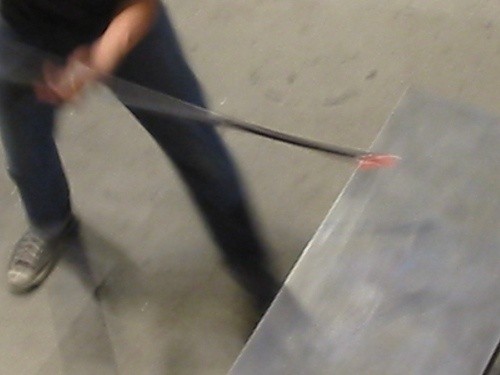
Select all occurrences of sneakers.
[7,214,83,293]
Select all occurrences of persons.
[0,0,281,322]
